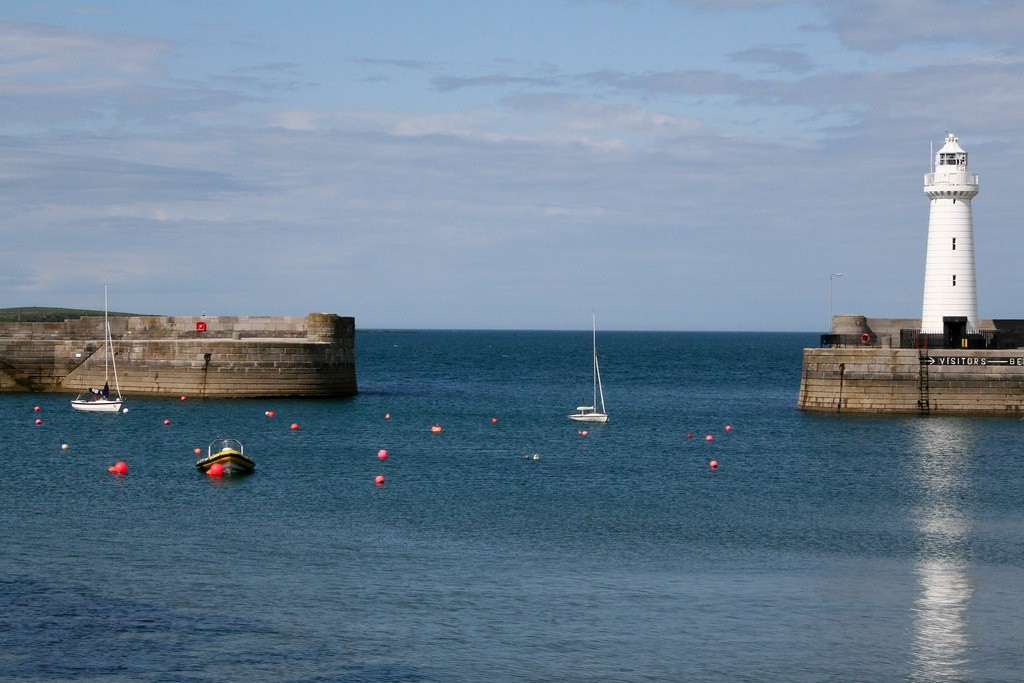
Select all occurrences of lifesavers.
[861,334,870,345]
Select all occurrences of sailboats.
[568,313,609,423]
[69,284,125,414]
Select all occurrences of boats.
[195,437,257,480]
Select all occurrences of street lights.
[829,272,845,333]
